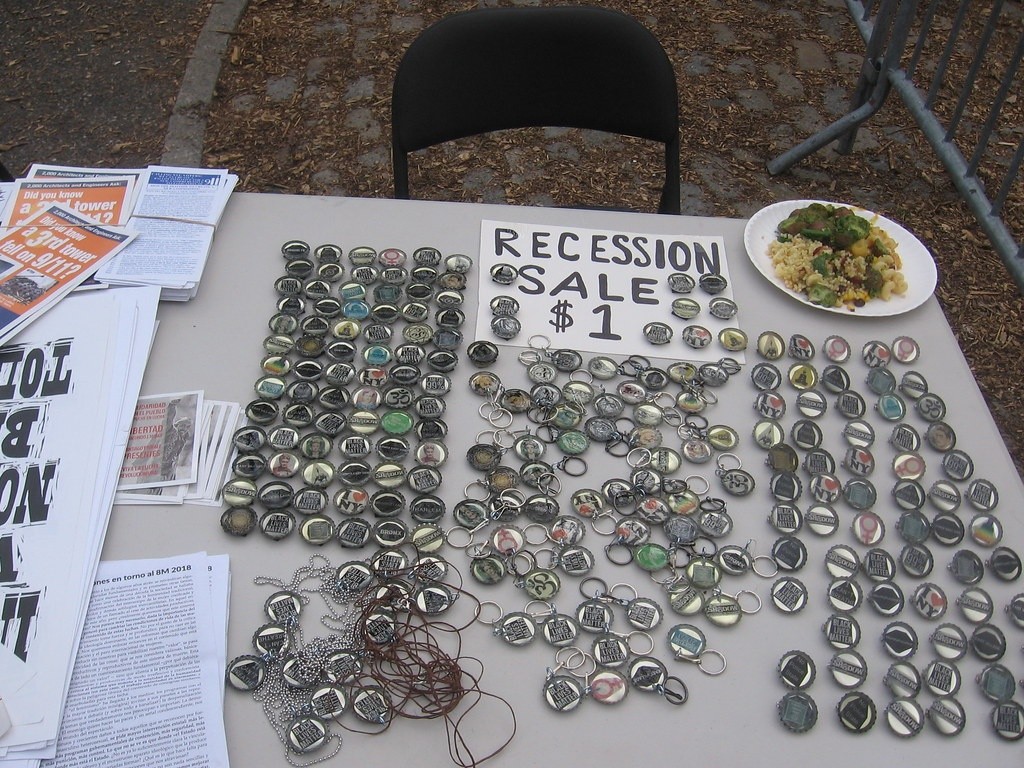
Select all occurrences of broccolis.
[778,203,907,307]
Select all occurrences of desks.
[74,191,1024,767]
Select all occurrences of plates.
[744,199,938,317]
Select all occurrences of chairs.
[392,5,682,217]
